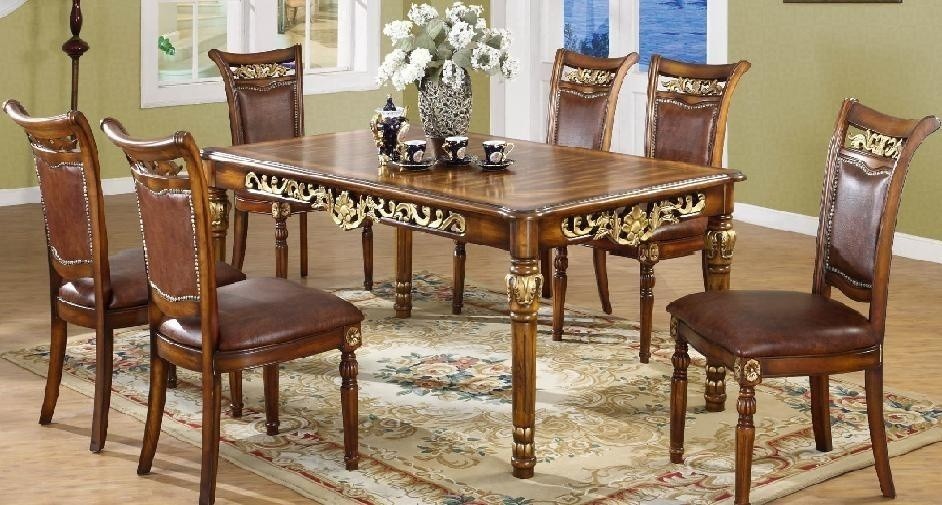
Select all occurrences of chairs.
[208,44,376,291]
[549,54,753,365]
[451,48,640,314]
[665,95,942,505]
[98,115,365,505]
[1,99,248,453]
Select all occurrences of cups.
[396,139,426,165]
[482,140,514,163]
[442,137,469,162]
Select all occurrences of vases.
[416,66,473,156]
[375,1,519,89]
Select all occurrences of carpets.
[0,269,942,505]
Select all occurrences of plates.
[392,159,436,171]
[434,154,478,167]
[476,159,515,171]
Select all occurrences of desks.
[199,120,748,479]
